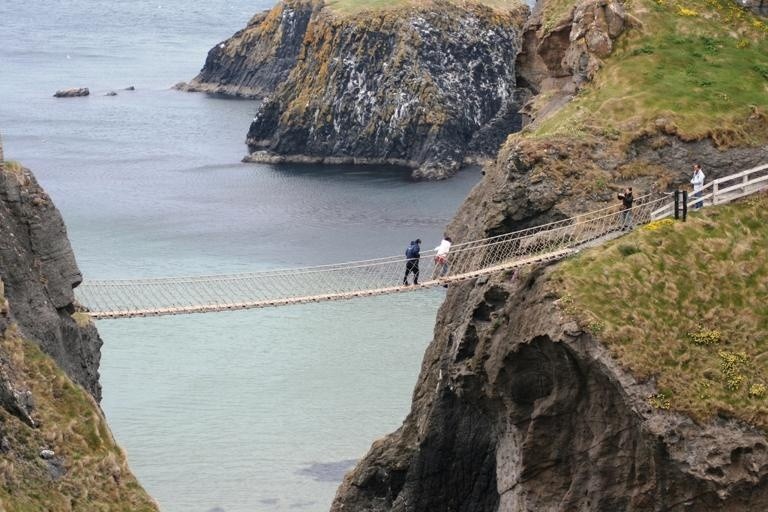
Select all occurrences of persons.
[690,164,706,208]
[618,187,633,231]
[432,237,452,280]
[403,239,422,286]
